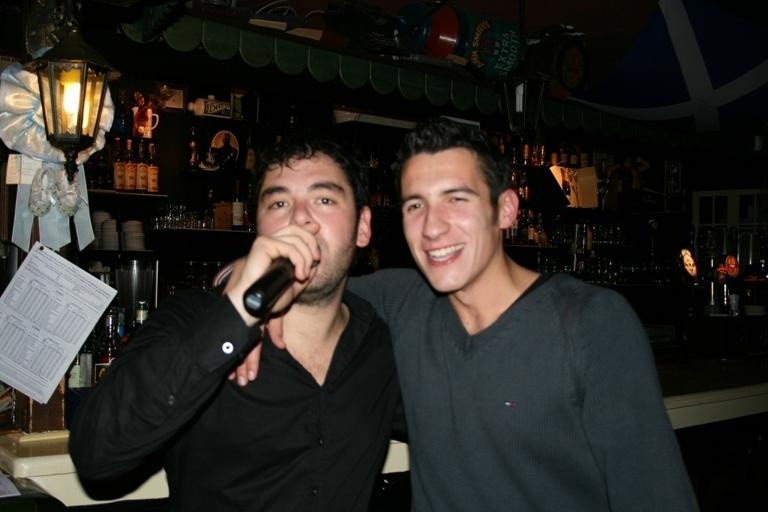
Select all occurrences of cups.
[744,305,765,316]
[115,260,230,328]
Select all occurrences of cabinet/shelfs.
[2,77,332,424]
[334,112,691,293]
[692,188,768,262]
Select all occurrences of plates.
[91,211,145,253]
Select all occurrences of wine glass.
[159,84,169,110]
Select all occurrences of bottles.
[66,301,149,389]
[85,94,633,279]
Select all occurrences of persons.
[219,116,699,511]
[67,136,409,511]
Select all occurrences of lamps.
[24,0,115,182]
[496,1,551,146]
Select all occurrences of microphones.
[243,257,321,318]
